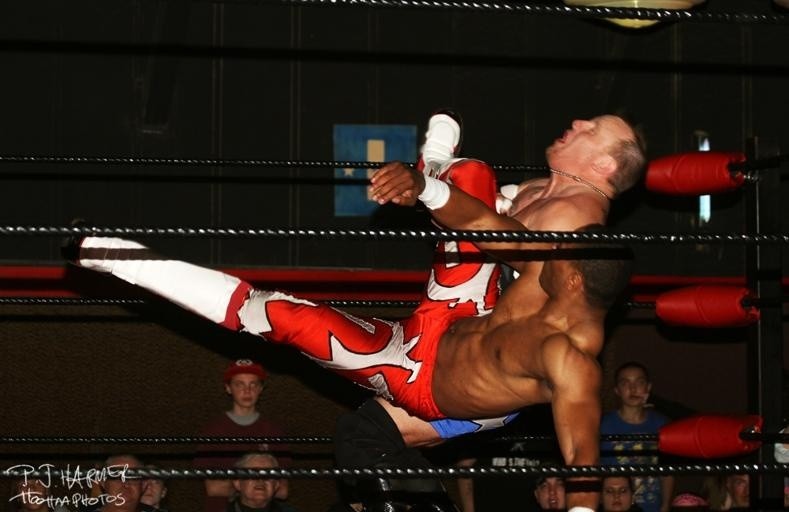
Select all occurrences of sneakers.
[415,106,465,192]
[59,211,111,278]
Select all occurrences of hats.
[224,358,267,384]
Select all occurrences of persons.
[50,106,647,512]
[5,477,50,511]
[673,466,753,510]
[597,360,677,469]
